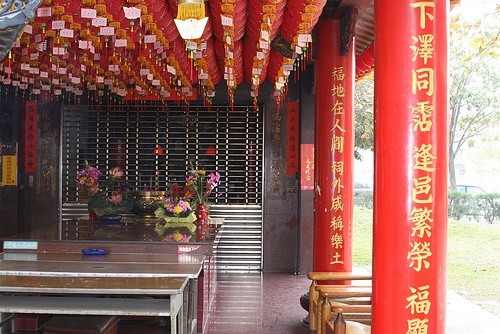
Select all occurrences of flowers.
[75,163,122,213]
[185,163,218,204]
[163,231,191,242]
[159,183,192,213]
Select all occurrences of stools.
[43,315,118,334]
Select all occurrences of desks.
[0,218,226,334]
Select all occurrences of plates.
[81,248,111,255]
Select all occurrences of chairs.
[306,270,371,334]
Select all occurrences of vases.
[154,208,197,223]
[99,213,121,222]
[195,204,208,219]
